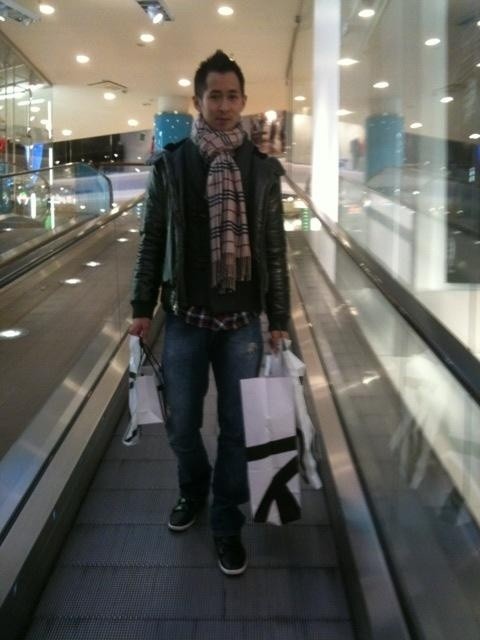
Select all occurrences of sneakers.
[213,532,247,576]
[168,499,205,530]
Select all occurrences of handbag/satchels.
[238,348,300,528]
[135,364,166,425]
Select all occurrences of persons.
[128,48,291,577]
